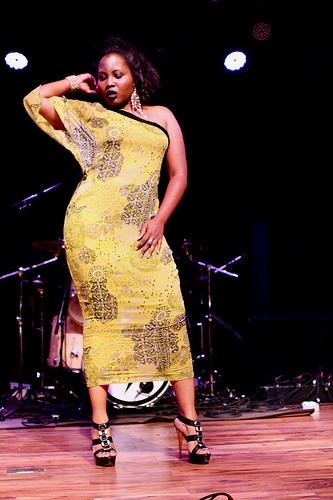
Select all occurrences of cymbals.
[33,241,65,253]
[22,279,62,288]
[169,242,221,256]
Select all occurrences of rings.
[147,239,153,244]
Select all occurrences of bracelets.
[65,74,80,91]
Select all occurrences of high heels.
[90,419,116,466]
[173,415,211,464]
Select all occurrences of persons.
[22,38,212,467]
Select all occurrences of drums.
[105,378,170,410]
[48,311,84,370]
[68,280,84,326]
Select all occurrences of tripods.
[184,255,243,406]
[1,253,82,420]
